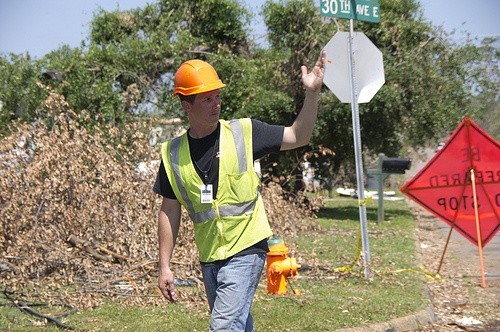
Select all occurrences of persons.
[152,48,328,332]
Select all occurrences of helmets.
[173,59,227,97]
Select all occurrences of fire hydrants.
[266,232,302,297]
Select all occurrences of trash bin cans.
[366,168,380,191]
